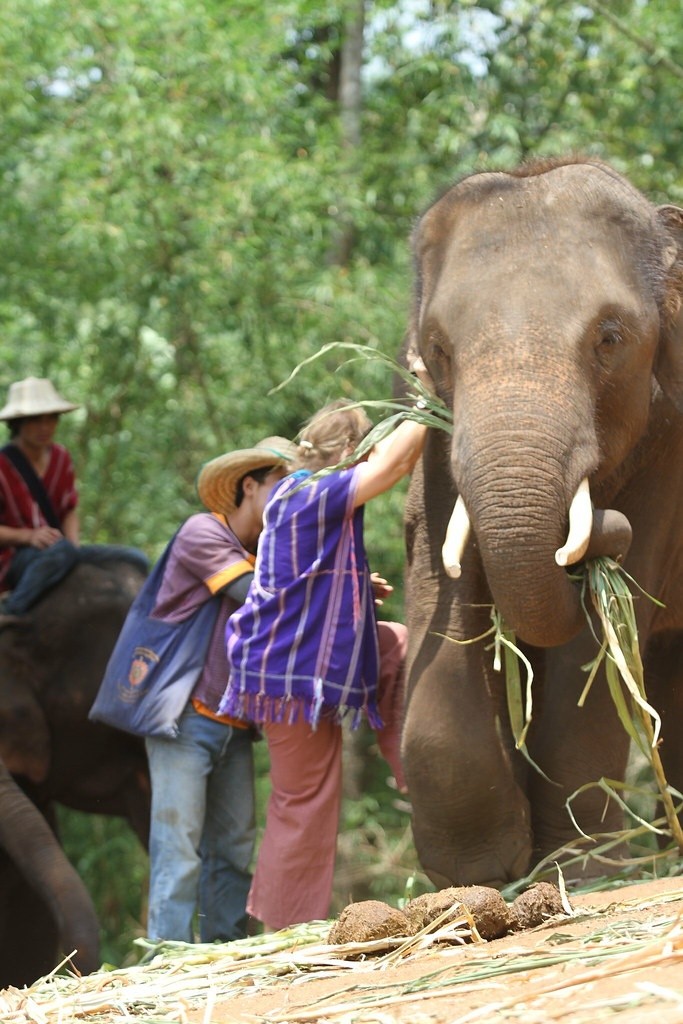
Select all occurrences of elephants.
[400,164,683,899]
[1,540,151,986]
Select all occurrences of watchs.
[415,397,432,410]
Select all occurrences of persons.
[145,435,394,966]
[219,358,437,937]
[0,377,149,626]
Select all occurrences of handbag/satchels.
[87,529,224,738]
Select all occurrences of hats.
[0,377,77,421]
[196,436,300,519]
